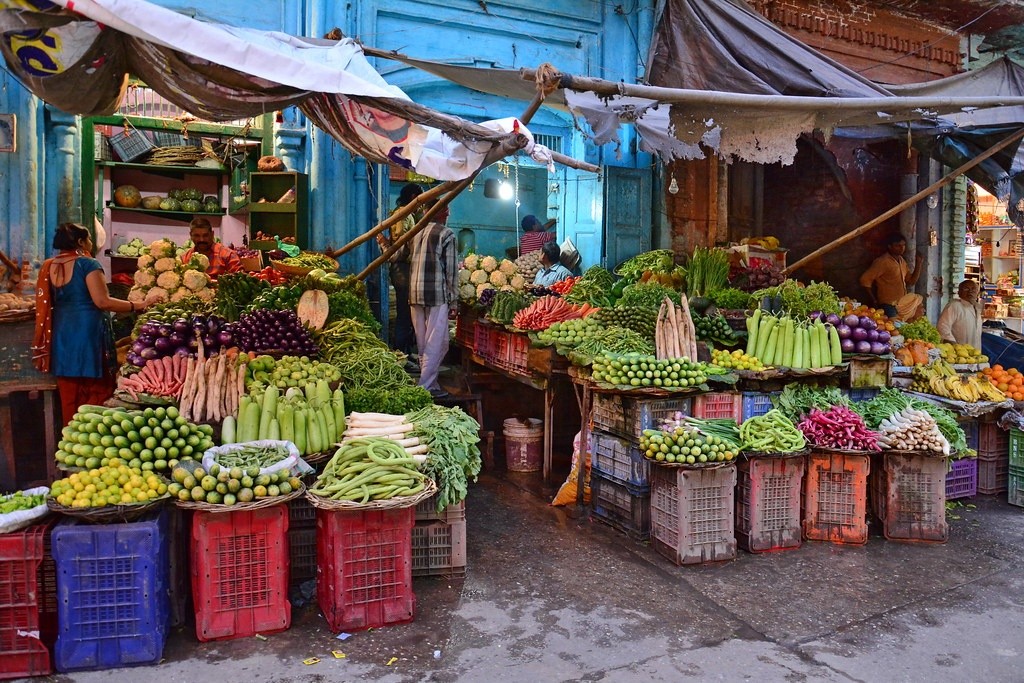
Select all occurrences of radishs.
[769,382,977,474]
[330,404,483,514]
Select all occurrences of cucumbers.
[54,403,216,469]
[639,428,739,464]
[595,303,747,345]
[590,350,707,387]
[168,465,302,505]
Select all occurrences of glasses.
[959,287,979,292]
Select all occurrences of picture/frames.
[0,113,17,153]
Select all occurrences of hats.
[892,292,923,323]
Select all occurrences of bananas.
[910,359,1007,405]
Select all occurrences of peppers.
[240,285,302,313]
[561,264,635,307]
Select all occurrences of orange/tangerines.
[976,364,1024,403]
[51,466,168,508]
[711,349,766,375]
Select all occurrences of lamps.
[668,171,679,195]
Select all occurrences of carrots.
[116,354,187,402]
[512,295,602,331]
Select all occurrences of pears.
[935,343,988,363]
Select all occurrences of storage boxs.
[238,249,264,272]
[0,295,1023,679]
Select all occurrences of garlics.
[656,409,749,451]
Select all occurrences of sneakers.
[430,389,448,399]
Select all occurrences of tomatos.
[247,265,289,285]
[547,275,583,295]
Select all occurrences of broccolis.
[454,253,525,300]
[118,237,219,305]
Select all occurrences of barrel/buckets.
[503,418,542,472]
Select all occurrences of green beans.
[320,315,412,390]
[216,445,290,468]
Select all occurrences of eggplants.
[807,311,892,356]
[126,307,319,366]
[527,284,560,298]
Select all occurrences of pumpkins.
[113,183,221,213]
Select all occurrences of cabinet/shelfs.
[248,170,310,251]
[96,160,228,217]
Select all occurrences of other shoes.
[407,352,417,363]
[403,361,421,373]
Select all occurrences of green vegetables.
[617,244,751,309]
[344,386,435,415]
[327,290,382,332]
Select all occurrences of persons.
[179,218,244,291]
[937,280,984,356]
[33,222,162,433]
[387,183,426,374]
[858,232,925,317]
[375,197,460,402]
[519,214,558,259]
[533,242,575,290]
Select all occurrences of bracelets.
[127,301,135,313]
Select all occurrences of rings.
[376,236,378,239]
